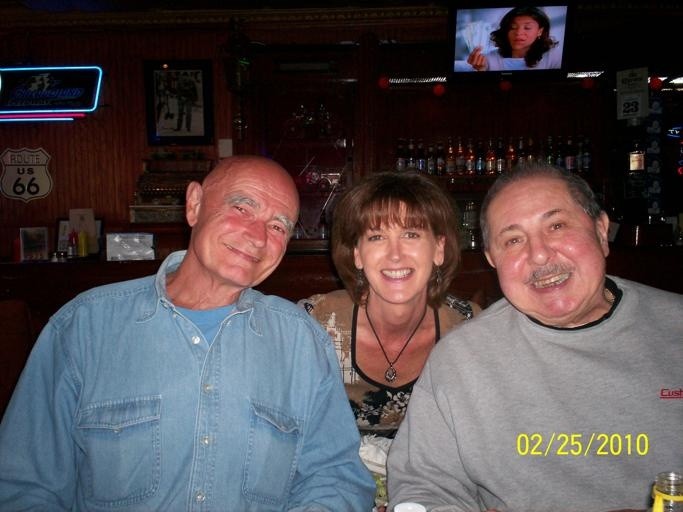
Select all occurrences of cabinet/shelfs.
[362,54,683,271]
[226,36,362,273]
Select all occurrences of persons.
[299,168,483,447]
[466,7,562,70]
[385,161,683,512]
[0,152,378,512]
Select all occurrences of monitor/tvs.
[448,0,571,73]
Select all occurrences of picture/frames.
[19,226,48,262]
[54,218,104,257]
[143,56,216,145]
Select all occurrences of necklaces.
[365,292,427,382]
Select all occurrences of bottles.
[628,118,645,175]
[50,228,87,263]
[461,200,480,249]
[394,133,591,178]
